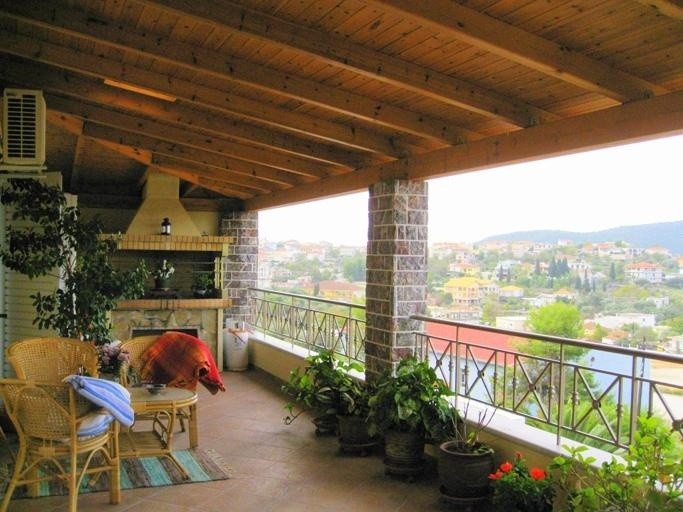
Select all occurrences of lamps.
[158,216,172,237]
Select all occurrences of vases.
[154,280,172,289]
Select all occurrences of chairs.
[0,332,200,511]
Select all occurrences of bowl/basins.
[145,383,166,395]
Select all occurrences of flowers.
[151,259,178,279]
[485,448,559,512]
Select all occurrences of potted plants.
[190,272,213,298]
[276,348,500,511]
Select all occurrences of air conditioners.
[0,87,47,172]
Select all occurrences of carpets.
[0,447,232,502]
